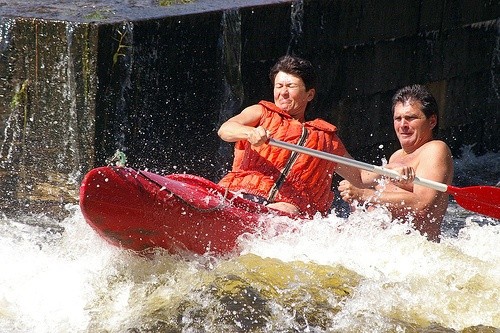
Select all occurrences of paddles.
[266,136,496,218]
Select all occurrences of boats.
[80,166,313,255]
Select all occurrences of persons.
[216,52,415,236]
[332,82,455,243]
